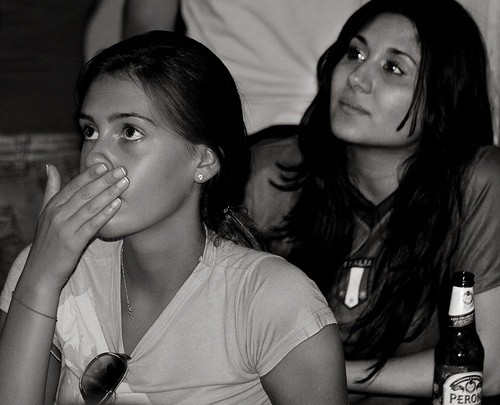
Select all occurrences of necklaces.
[120,250,142,340]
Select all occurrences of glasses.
[79,352,131,405]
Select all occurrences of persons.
[0,29,352,405]
[122,0,500,405]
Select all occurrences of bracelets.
[12,291,57,322]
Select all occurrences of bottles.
[433,271,485,405]
